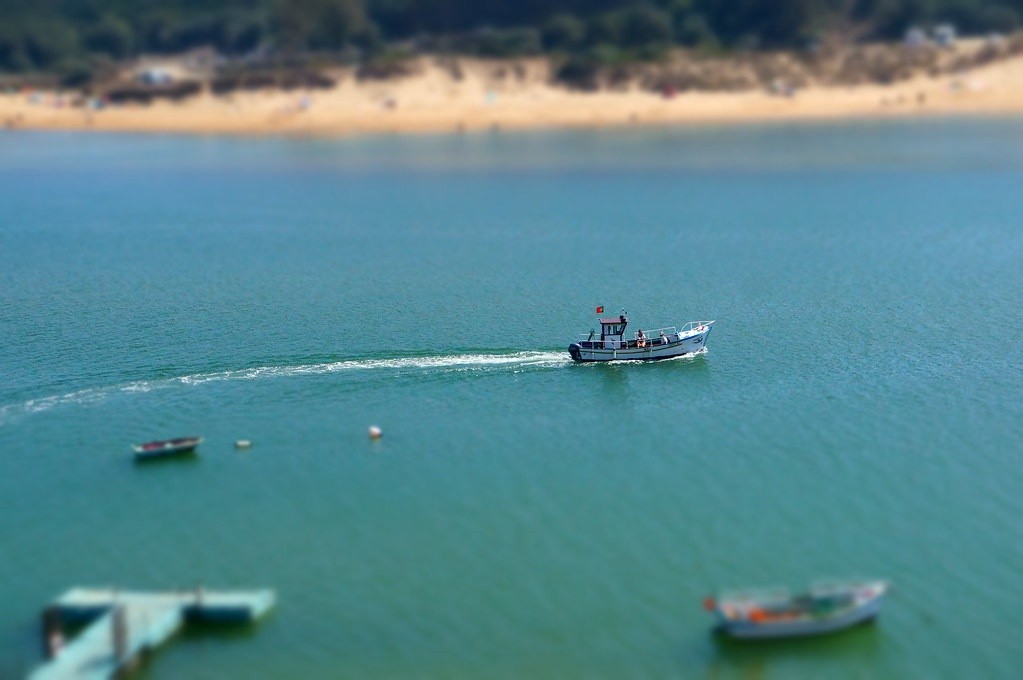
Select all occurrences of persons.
[660,331,668,344]
[636,329,646,348]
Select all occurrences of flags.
[596,305,604,313]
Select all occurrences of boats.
[708,576,891,637]
[567,311,715,365]
[132,435,205,458]
